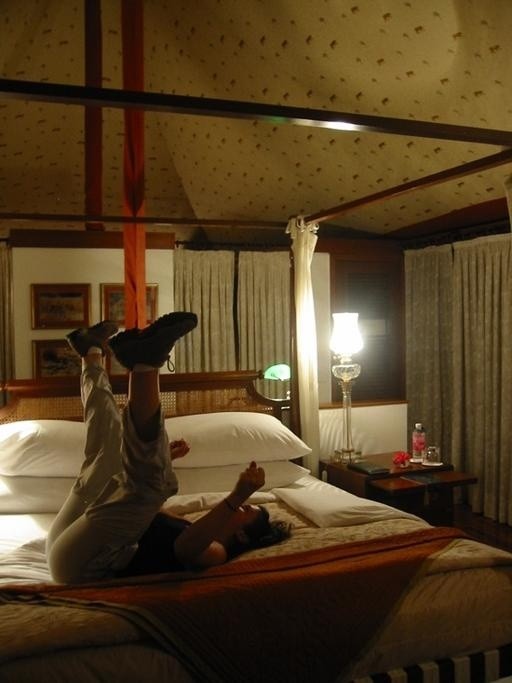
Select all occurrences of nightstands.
[318,451,478,527]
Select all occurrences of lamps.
[329,312,365,465]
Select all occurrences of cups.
[422,447,441,464]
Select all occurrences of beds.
[0,370,512,682]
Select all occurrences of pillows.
[0,420,89,477]
[0,476,79,515]
[172,460,311,495]
[165,411,313,468]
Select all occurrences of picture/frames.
[30,283,92,330]
[100,283,159,328]
[32,338,82,379]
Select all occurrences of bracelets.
[223,497,237,513]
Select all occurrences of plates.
[408,458,427,463]
[422,462,444,467]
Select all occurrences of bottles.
[412,423,425,459]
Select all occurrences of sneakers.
[64,317,117,356]
[110,312,201,371]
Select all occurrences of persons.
[44,311,293,583]
